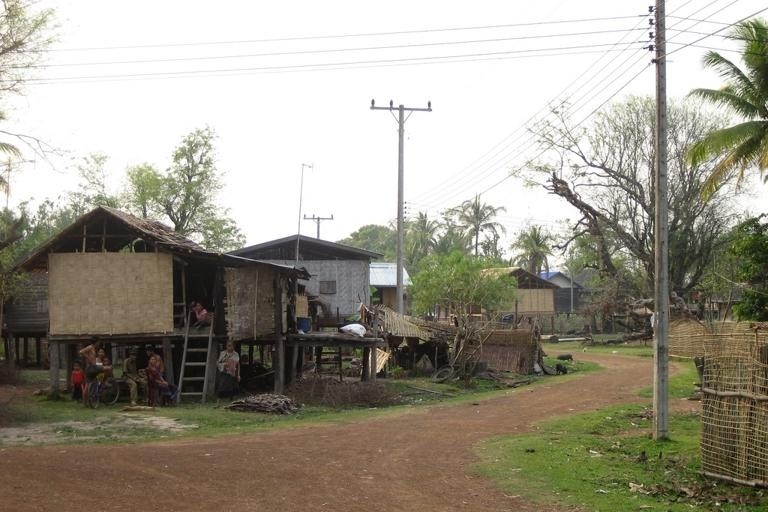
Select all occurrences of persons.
[190,301,208,333]
[212,339,242,408]
[70,336,180,409]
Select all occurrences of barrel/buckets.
[296,315,314,334]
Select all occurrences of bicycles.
[86,371,120,410]
